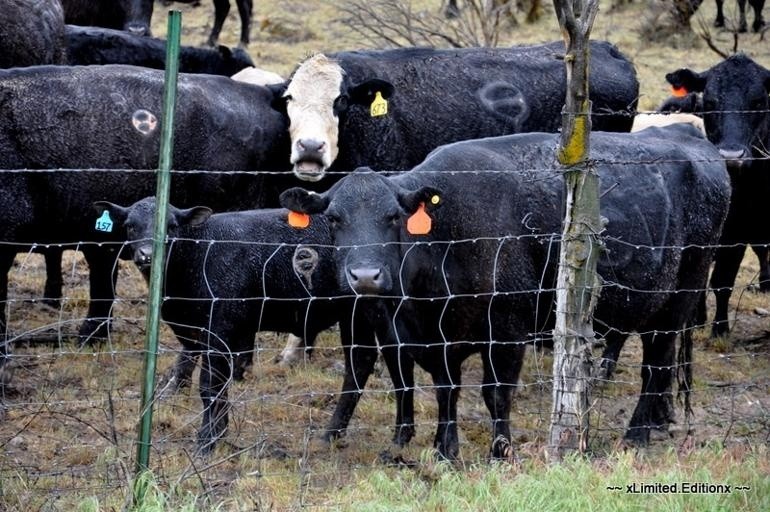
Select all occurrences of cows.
[1,0,770,512]
